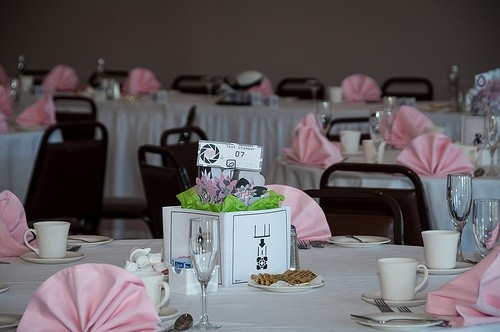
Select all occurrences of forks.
[298,239,329,249]
[375,299,449,328]
[68,245,81,252]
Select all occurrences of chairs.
[17,70,435,247]
[16,69,51,84]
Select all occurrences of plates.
[357,313,445,328]
[66,235,113,247]
[362,294,426,307]
[342,150,365,155]
[0,283,10,294]
[246,278,323,294]
[417,262,470,272]
[158,306,179,321]
[20,252,84,263]
[323,234,392,247]
[0,313,23,330]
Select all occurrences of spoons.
[169,315,193,332]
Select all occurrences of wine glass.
[190,217,222,330]
[317,95,399,164]
[484,114,500,178]
[447,173,472,263]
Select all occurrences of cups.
[420,229,461,270]
[471,198,500,263]
[24,221,70,257]
[361,138,385,164]
[338,130,362,152]
[329,86,342,101]
[132,272,171,314]
[377,258,429,300]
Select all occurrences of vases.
[162,206,292,288]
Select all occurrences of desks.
[1,90,500,239]
[1,240,500,332]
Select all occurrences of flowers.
[177,171,285,212]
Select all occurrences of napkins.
[39,65,79,92]
[283,160,500,252]
[0,190,37,258]
[122,68,160,94]
[265,184,332,241]
[427,222,500,327]
[340,75,382,101]
[0,64,17,134]
[16,262,161,332]
[377,104,432,149]
[283,126,341,169]
[16,94,57,128]
[292,113,317,135]
[250,76,273,94]
[394,133,475,177]
